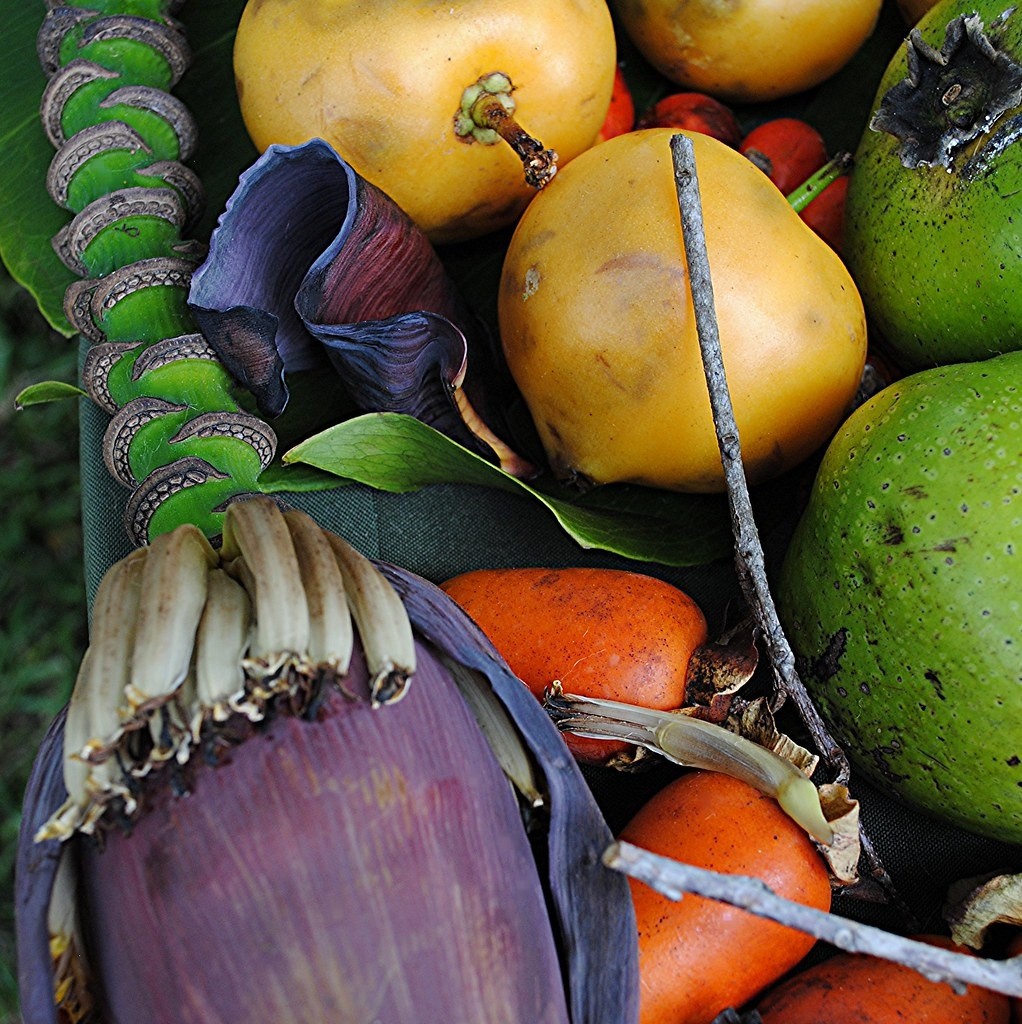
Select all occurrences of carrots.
[432,569,1016,1023]
